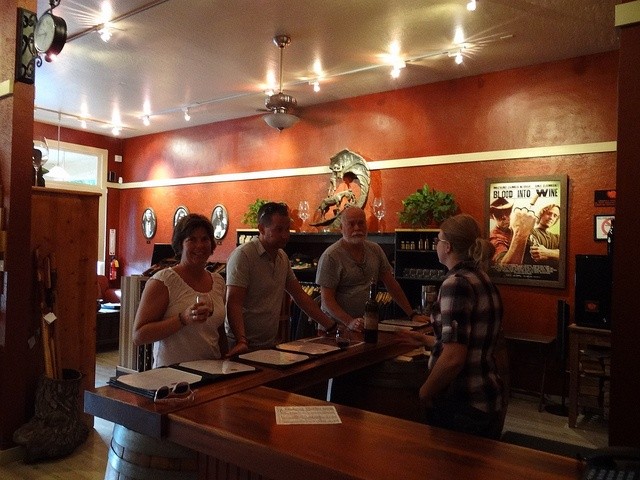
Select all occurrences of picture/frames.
[142,207,156,239]
[485,174,567,288]
[593,189,617,207]
[172,204,190,231]
[210,205,228,240]
[593,215,615,241]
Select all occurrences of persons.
[512,204,560,268]
[418,213,515,442]
[131,213,229,374]
[224,202,345,359]
[489,198,535,265]
[316,207,431,336]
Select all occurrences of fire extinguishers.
[110,256,117,280]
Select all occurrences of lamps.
[143,118,150,127]
[183,112,192,123]
[388,67,401,77]
[453,53,466,65]
[262,114,294,134]
[313,84,321,92]
[467,2,477,11]
[102,26,112,42]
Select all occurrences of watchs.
[409,308,423,317]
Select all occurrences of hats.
[490,198,514,220]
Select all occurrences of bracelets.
[325,322,337,333]
[177,312,186,327]
[235,334,248,345]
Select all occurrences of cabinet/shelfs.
[31,184,103,430]
[236,230,397,273]
[568,323,613,428]
[395,228,448,319]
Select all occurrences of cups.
[196,294,214,317]
[336,325,350,349]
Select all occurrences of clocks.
[33,13,55,54]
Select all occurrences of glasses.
[154,380,198,403]
[434,236,449,245]
[259,202,288,221]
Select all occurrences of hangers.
[295,281,395,305]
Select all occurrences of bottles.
[363,277,379,344]
[418,239,423,249]
[405,241,410,250]
[424,238,429,250]
[410,241,415,250]
[432,237,438,250]
[400,241,405,250]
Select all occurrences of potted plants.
[240,199,269,228]
[400,184,460,229]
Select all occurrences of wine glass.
[373,197,386,232]
[298,200,309,232]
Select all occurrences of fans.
[243,91,341,128]
